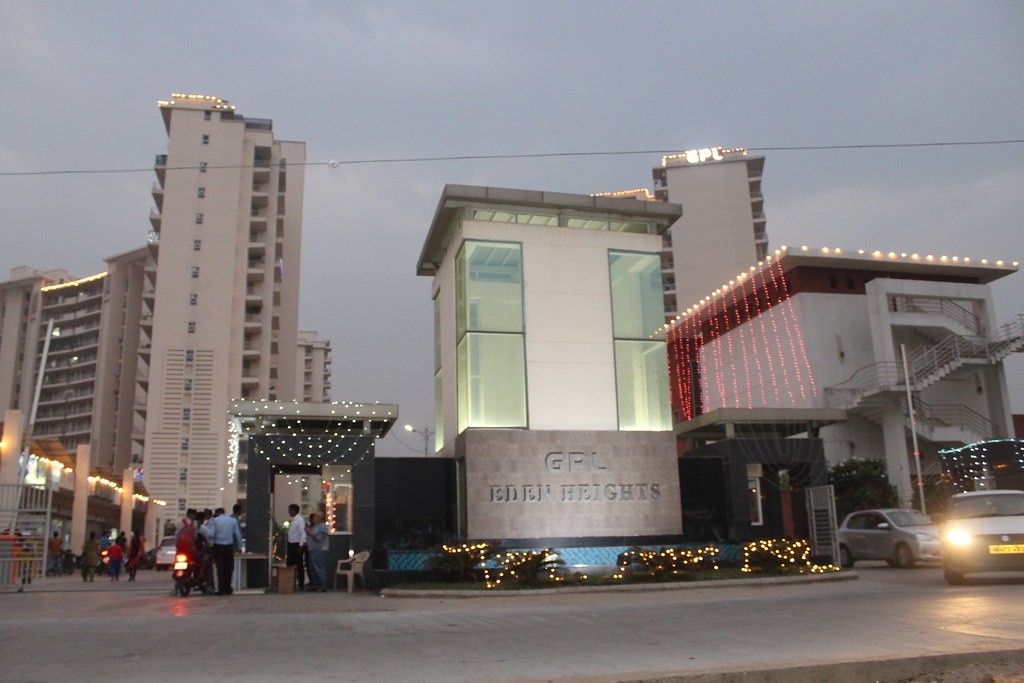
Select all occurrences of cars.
[931,490,1023,582]
[155,535,181,570]
[837,508,944,570]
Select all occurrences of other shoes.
[80,570,139,583]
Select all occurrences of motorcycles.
[172,543,215,598]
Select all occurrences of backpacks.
[177,519,196,551]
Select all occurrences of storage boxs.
[278,568,296,593]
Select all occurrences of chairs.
[333,550,370,594]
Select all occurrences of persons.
[305,511,328,595]
[19,501,248,601]
[285,505,312,593]
